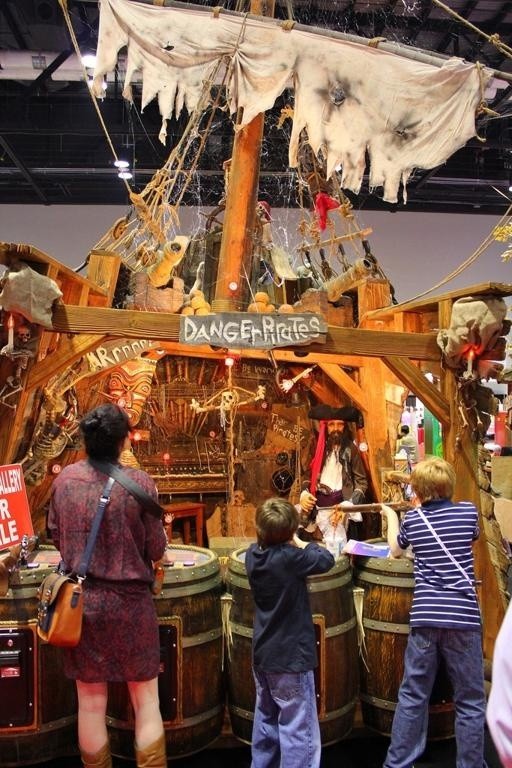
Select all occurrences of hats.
[308,404,363,422]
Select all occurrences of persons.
[293,403,370,543]
[480,591,512,768]
[243,497,335,768]
[43,404,170,768]
[375,456,490,768]
[398,424,419,464]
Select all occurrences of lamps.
[113,151,133,180]
[80,50,108,92]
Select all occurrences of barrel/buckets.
[354,541,455,752]
[1,544,75,756]
[107,545,224,764]
[224,548,354,755]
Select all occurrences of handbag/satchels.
[36,572,83,647]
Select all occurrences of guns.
[317,501,415,513]
